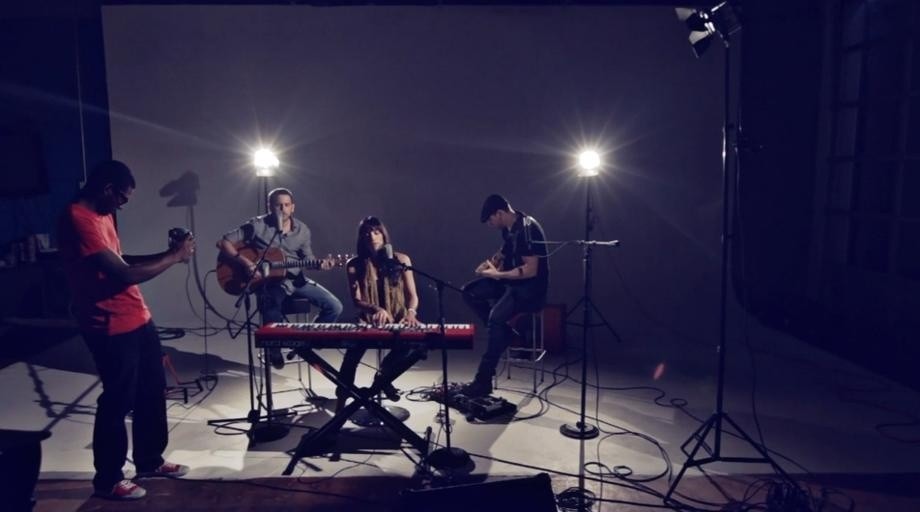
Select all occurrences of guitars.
[216,240,356,296]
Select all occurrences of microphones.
[382,244,397,286]
[274,210,284,233]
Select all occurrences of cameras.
[168,227,191,257]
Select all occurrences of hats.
[481,194,508,224]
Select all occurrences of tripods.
[207,234,299,450]
[662,43,811,507]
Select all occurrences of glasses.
[112,186,128,206]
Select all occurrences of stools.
[257,292,320,413]
[352,347,409,427]
[494,299,550,392]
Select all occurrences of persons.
[464,193,547,395]
[337,216,419,416]
[69,160,194,499]
[221,187,343,372]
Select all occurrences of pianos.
[255,320,476,349]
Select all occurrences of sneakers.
[109,479,146,499]
[136,461,189,478]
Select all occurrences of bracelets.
[405,307,419,316]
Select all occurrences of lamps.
[675,0,769,81]
[255,149,280,222]
[579,150,602,239]
